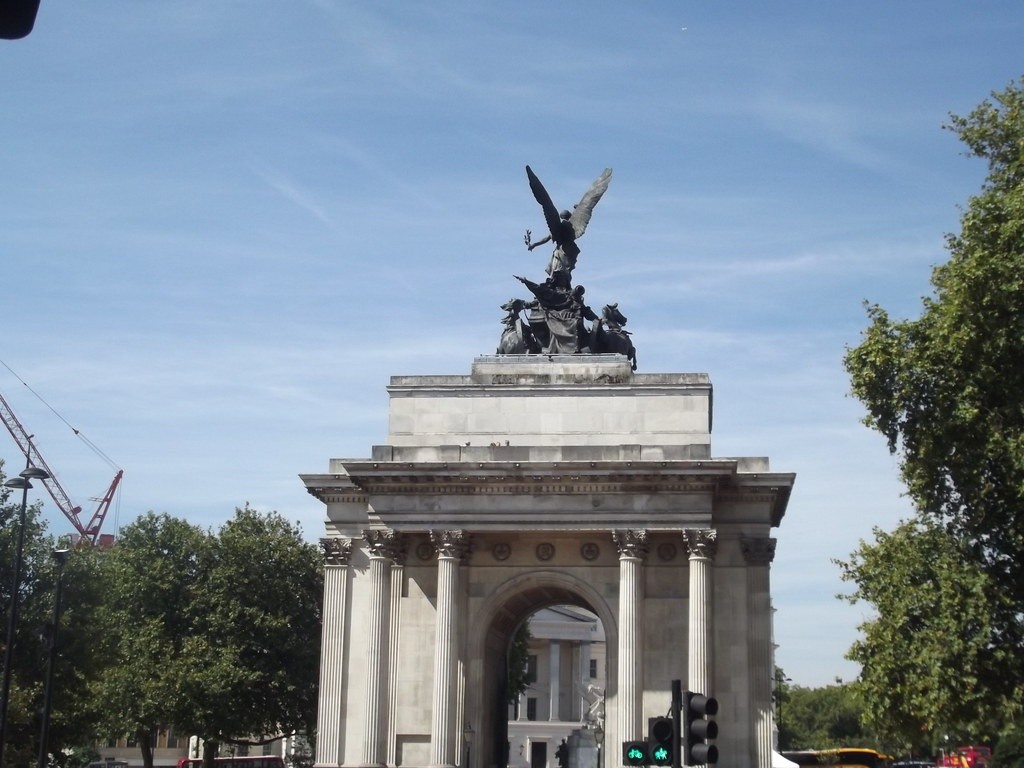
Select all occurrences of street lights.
[0,468,50,768]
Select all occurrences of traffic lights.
[683,691,719,766]
[622,717,672,766]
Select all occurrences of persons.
[498,297,538,352]
[590,300,639,358]
[512,272,599,358]
[524,209,581,277]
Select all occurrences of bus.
[937,745,992,768]
[782,748,894,768]
